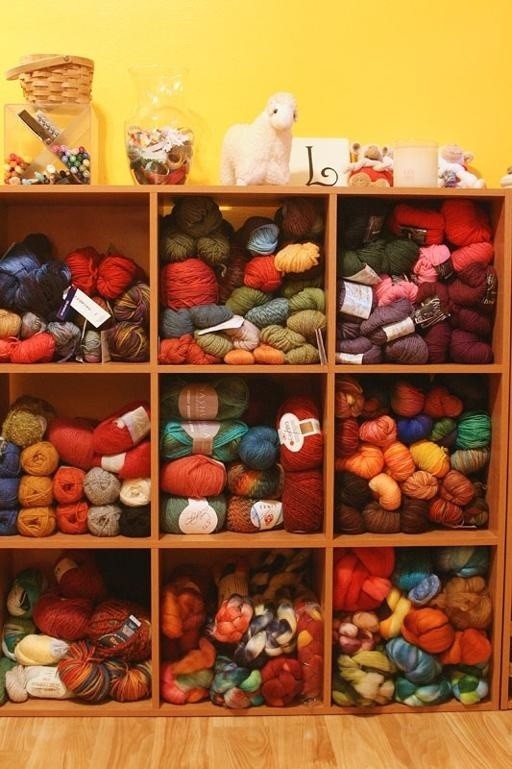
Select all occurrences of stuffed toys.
[219,91,299,186]
[438,145,486,188]
[349,141,393,189]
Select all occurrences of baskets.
[6,54,95,115]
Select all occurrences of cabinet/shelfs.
[2,185,511,720]
